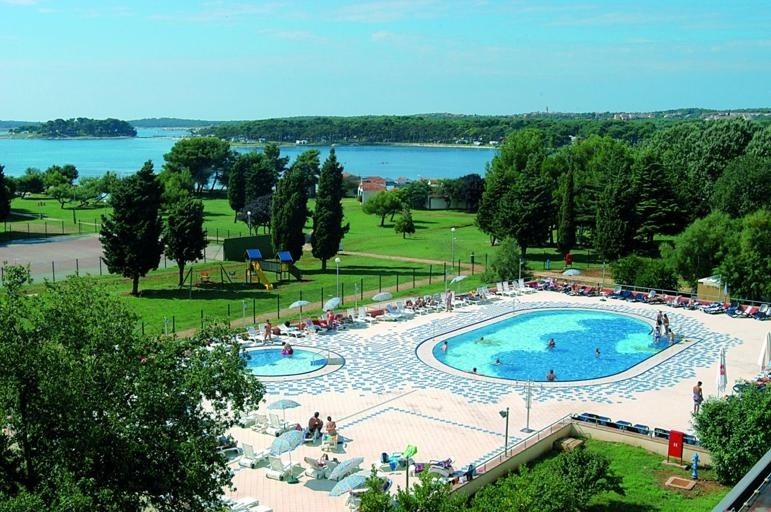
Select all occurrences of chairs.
[461,287,501,306]
[495,278,535,298]
[343,450,476,508]
[536,276,770,322]
[572,410,697,446]
[215,411,340,482]
[383,290,466,322]
[229,306,376,345]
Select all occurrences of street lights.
[335,258,341,297]
[247,211,252,236]
[450,228,456,267]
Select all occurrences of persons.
[595,348,601,358]
[440,341,449,353]
[597,282,601,295]
[446,290,453,312]
[326,310,334,330]
[693,381,704,416]
[546,370,558,381]
[281,341,294,355]
[262,318,273,344]
[497,359,501,365]
[405,296,435,312]
[549,338,555,350]
[308,412,323,436]
[326,416,337,436]
[472,368,478,373]
[652,310,675,346]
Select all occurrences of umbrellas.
[329,471,369,496]
[267,398,302,429]
[328,455,366,481]
[718,347,728,399]
[448,274,467,287]
[322,298,340,313]
[370,292,393,302]
[767,331,771,373]
[271,429,305,475]
[722,282,728,305]
[289,299,310,310]
[564,268,581,277]
